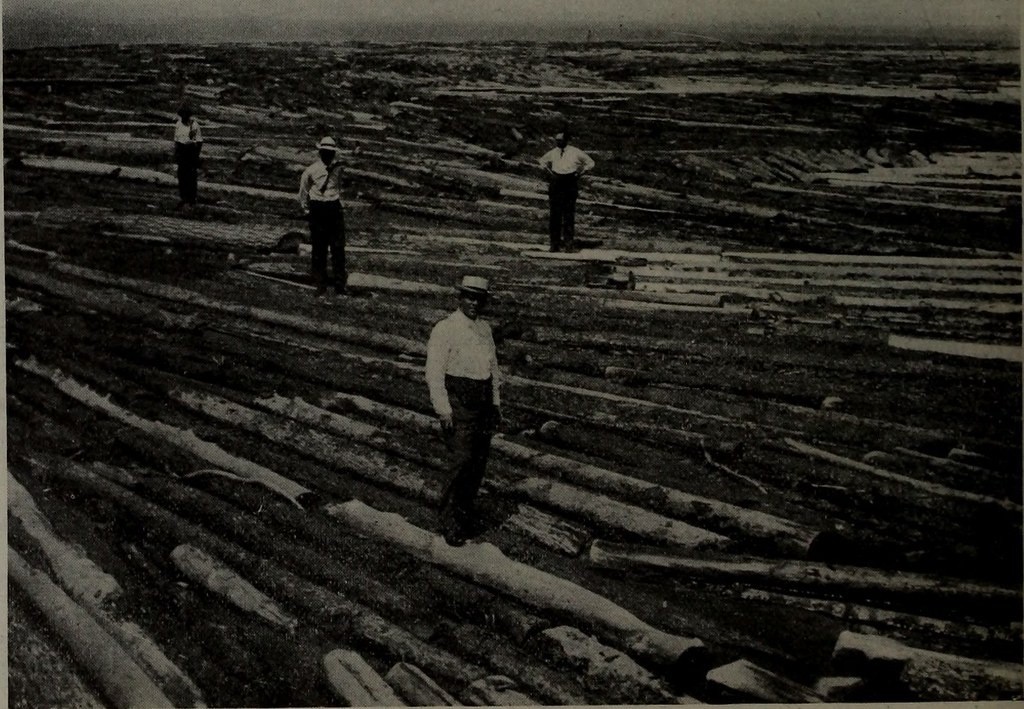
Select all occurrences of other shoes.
[314,285,327,297]
[446,534,464,547]
[334,287,348,296]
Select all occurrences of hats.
[315,135,338,152]
[177,102,194,118]
[454,275,495,298]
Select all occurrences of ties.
[319,166,335,194]
[561,149,564,157]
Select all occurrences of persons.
[538,132,596,250]
[174,101,205,201]
[424,275,504,548]
[298,136,349,288]
